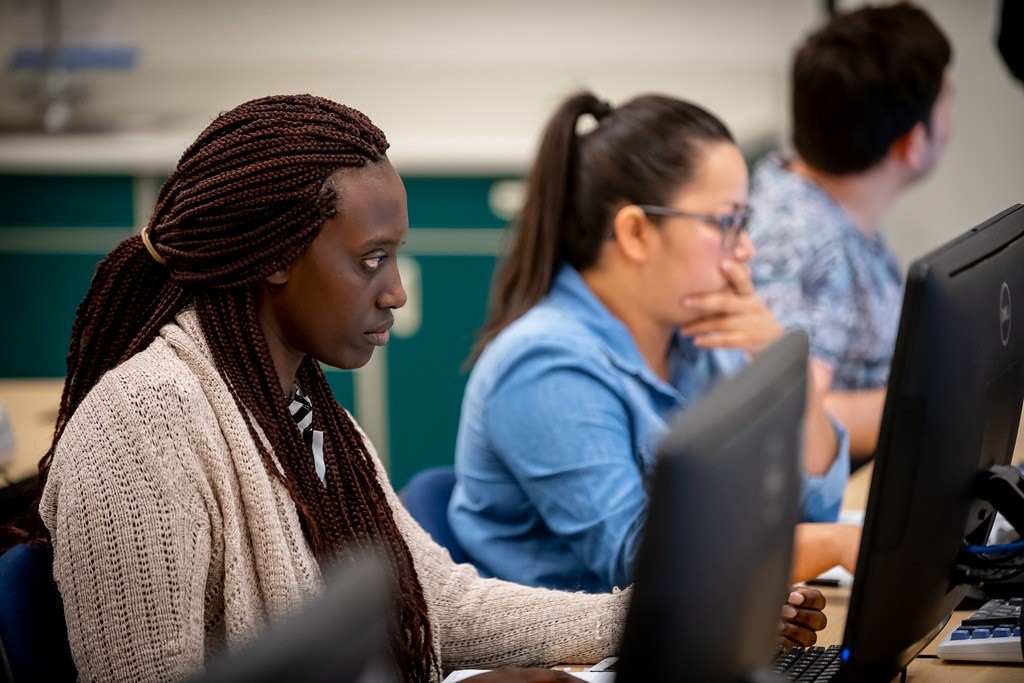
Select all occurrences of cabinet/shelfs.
[0,125,542,495]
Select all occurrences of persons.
[451,93,867,598]
[739,2,959,464]
[36,93,829,683]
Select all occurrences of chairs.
[0,535,75,682]
[398,464,472,568]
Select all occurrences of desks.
[544,405,1024,683]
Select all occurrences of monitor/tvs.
[614,326,813,683]
[169,549,400,683]
[838,200,1024,683]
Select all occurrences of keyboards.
[766,643,842,683]
[938,596,1024,662]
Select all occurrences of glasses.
[608,205,754,250]
